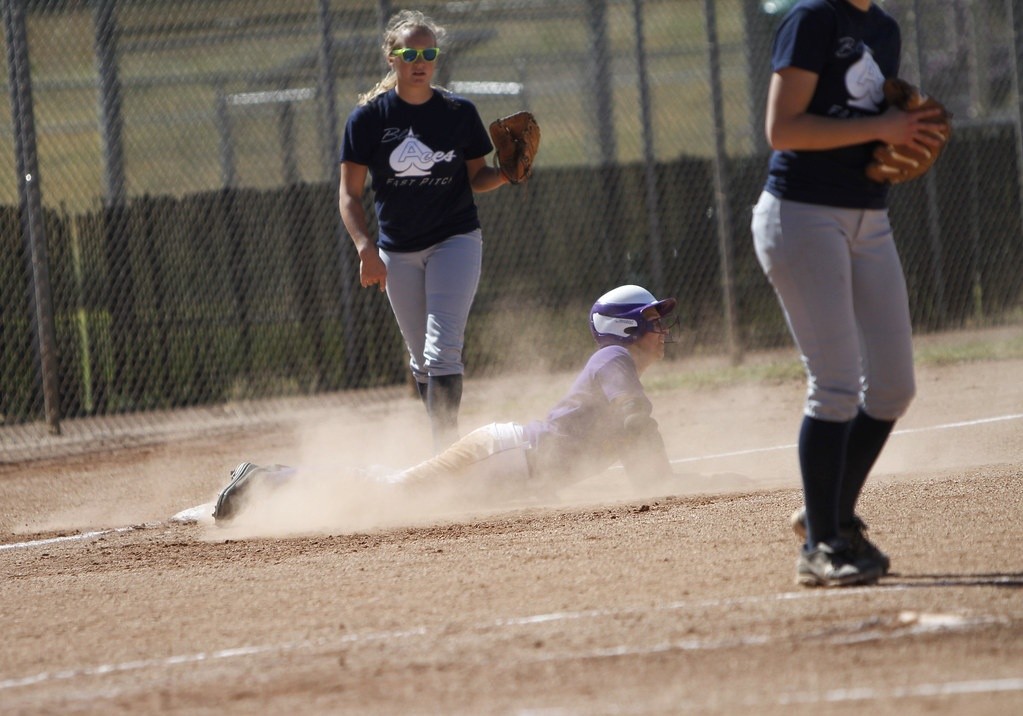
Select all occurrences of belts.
[523,422,540,482]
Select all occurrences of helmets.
[588,284,677,348]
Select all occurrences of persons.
[751,0,953,587]
[337,10,539,447]
[214,286,678,527]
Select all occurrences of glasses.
[391,48,440,64]
[646,318,665,335]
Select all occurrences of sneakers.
[797,536,883,586]
[792,503,889,570]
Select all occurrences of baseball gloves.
[486,110,541,188]
[868,78,951,186]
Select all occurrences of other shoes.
[212,462,266,526]
